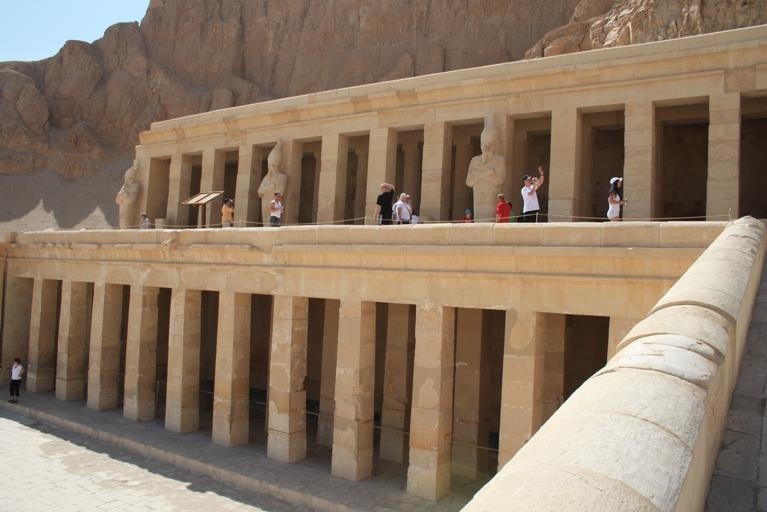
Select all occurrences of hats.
[521,175,532,180]
[610,177,623,185]
[400,193,410,200]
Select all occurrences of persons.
[8,358,25,403]
[496,193,515,223]
[270,191,288,227]
[221,198,234,227]
[521,166,544,222]
[141,213,151,229]
[607,177,628,221]
[372,183,417,225]
[463,208,474,223]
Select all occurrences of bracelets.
[540,174,544,176]
